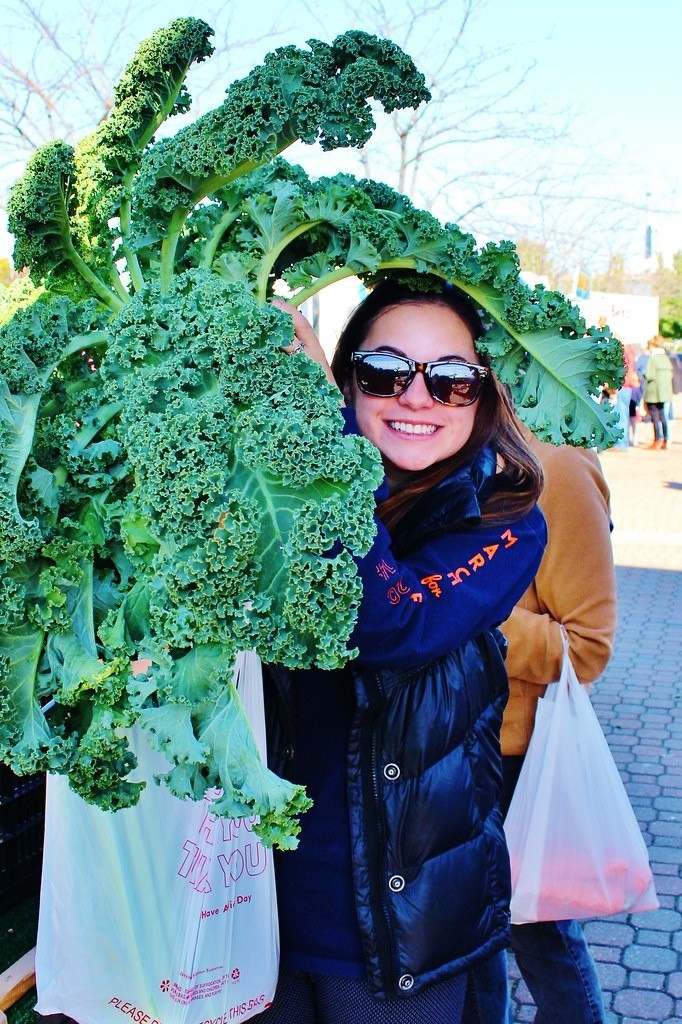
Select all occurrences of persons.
[464,408,619,1024]
[611,334,682,449]
[250,276,544,1024]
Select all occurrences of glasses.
[350,351,485,408]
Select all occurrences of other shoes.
[661,439,667,449]
[643,440,660,450]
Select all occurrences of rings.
[286,342,304,357]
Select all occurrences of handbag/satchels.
[501,625,659,925]
[36,647,283,1022]
